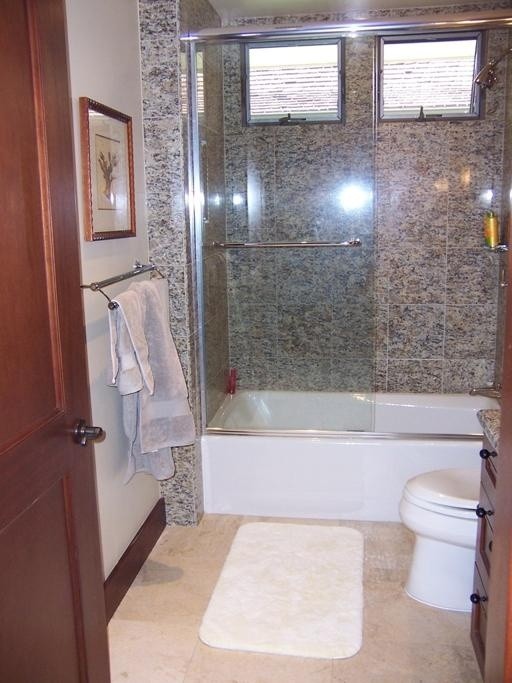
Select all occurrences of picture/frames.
[77,95,137,241]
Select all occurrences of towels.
[110,277,196,481]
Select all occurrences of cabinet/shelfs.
[470,431,505,683]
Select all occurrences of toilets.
[399,467,481,610]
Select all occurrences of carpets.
[199,519,365,661]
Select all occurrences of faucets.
[469,386,501,398]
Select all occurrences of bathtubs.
[200,387,502,523]
